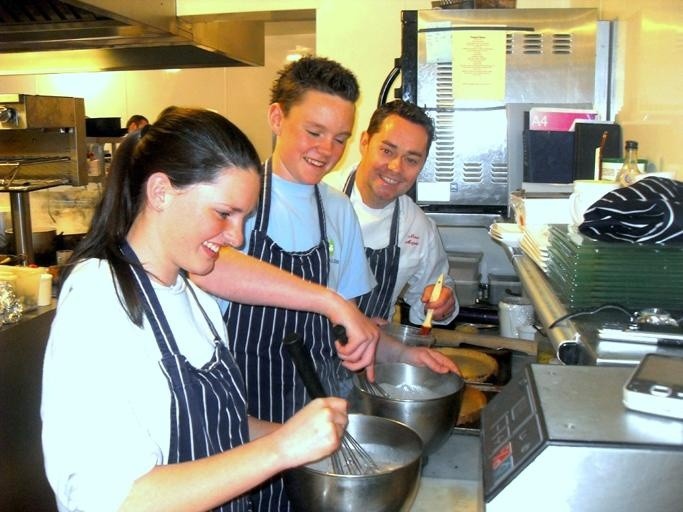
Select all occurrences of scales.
[477,363,683,512]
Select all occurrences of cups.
[36,273,54,307]
[54,250,73,265]
[377,320,435,348]
[516,324,542,341]
[568,179,618,229]
[496,295,535,338]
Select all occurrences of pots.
[0,225,63,255]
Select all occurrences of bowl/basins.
[283,413,425,511]
[348,360,466,464]
[85,117,121,136]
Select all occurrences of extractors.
[0,1,113,27]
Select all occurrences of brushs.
[419,273,445,335]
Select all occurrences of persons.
[319,96,462,398]
[37,105,353,512]
[186,52,466,511]
[121,112,150,134]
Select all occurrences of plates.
[456,385,488,428]
[432,345,500,380]
[487,221,520,246]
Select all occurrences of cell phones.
[622,352,683,420]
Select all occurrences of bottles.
[616,140,643,188]
